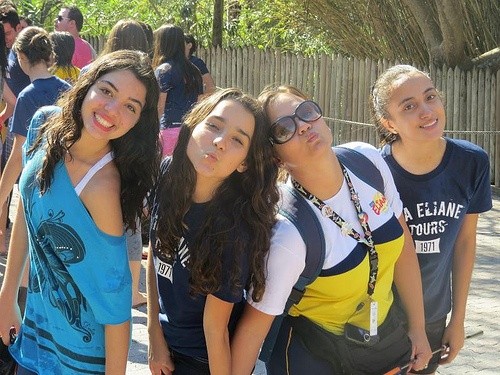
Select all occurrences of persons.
[0,49,163,375]
[0,0,216,308]
[231,82,432,375]
[146,88,283,375]
[369,64,492,375]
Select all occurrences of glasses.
[267,100,323,147]
[57,15,71,21]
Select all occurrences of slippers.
[132,292,147,308]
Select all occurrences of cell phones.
[344,323,380,348]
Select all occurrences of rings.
[425,363,428,368]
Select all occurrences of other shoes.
[141,252,148,258]
[0,248,8,256]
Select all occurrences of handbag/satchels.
[327,316,412,375]
